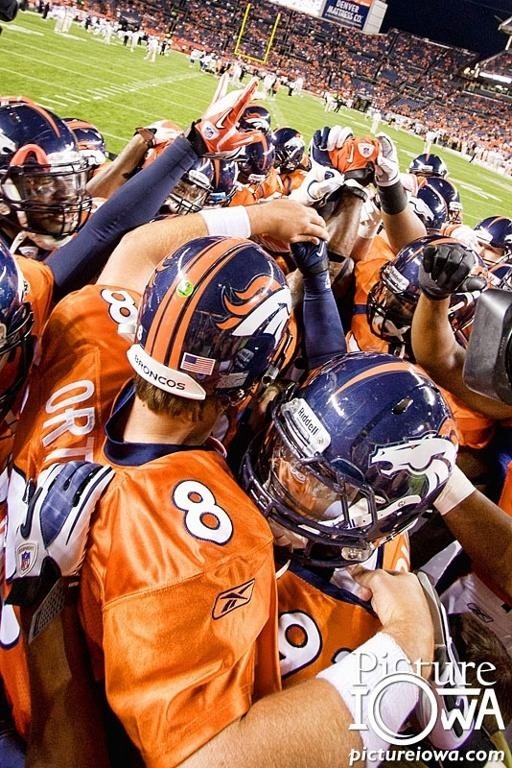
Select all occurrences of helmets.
[365,215,510,347]
[127,236,293,400]
[239,350,460,570]
[1,239,35,354]
[0,95,307,236]
[409,154,463,229]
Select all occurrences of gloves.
[300,123,406,218]
[19,461,116,611]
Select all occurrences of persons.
[1,0,510,767]
